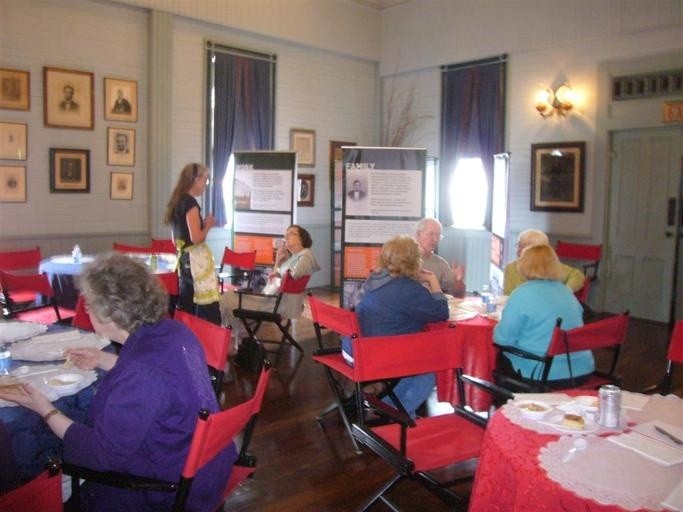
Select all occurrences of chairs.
[305,291,413,453]
[554,241,603,305]
[637,320,683,395]
[352,323,514,512]
[492,310,631,404]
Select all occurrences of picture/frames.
[297,174,314,207]
[109,171,134,201]
[0,122,28,163]
[103,77,138,124]
[0,165,28,204]
[290,130,316,167]
[530,141,585,213]
[0,69,30,111]
[49,148,90,193]
[43,67,94,130]
[106,127,136,167]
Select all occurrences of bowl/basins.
[47,373,84,391]
[576,396,600,413]
[514,400,553,420]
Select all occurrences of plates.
[547,410,600,433]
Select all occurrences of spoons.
[63,355,71,368]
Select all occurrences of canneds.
[599,384,622,429]
[149,254,157,270]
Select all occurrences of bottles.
[72,244,82,264]
[480,285,491,317]
[487,296,497,320]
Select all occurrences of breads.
[562,414,585,430]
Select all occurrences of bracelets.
[43,408,58,425]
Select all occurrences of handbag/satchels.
[237,338,268,370]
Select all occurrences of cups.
[150,254,158,271]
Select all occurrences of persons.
[115,134,129,154]
[1,247,238,512]
[415,215,467,293]
[111,89,130,113]
[162,163,226,418]
[504,230,585,296]
[58,84,78,112]
[220,224,321,331]
[492,244,596,405]
[341,234,450,420]
[349,180,364,199]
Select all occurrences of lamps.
[532,83,576,119]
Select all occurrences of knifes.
[654,424,683,445]
